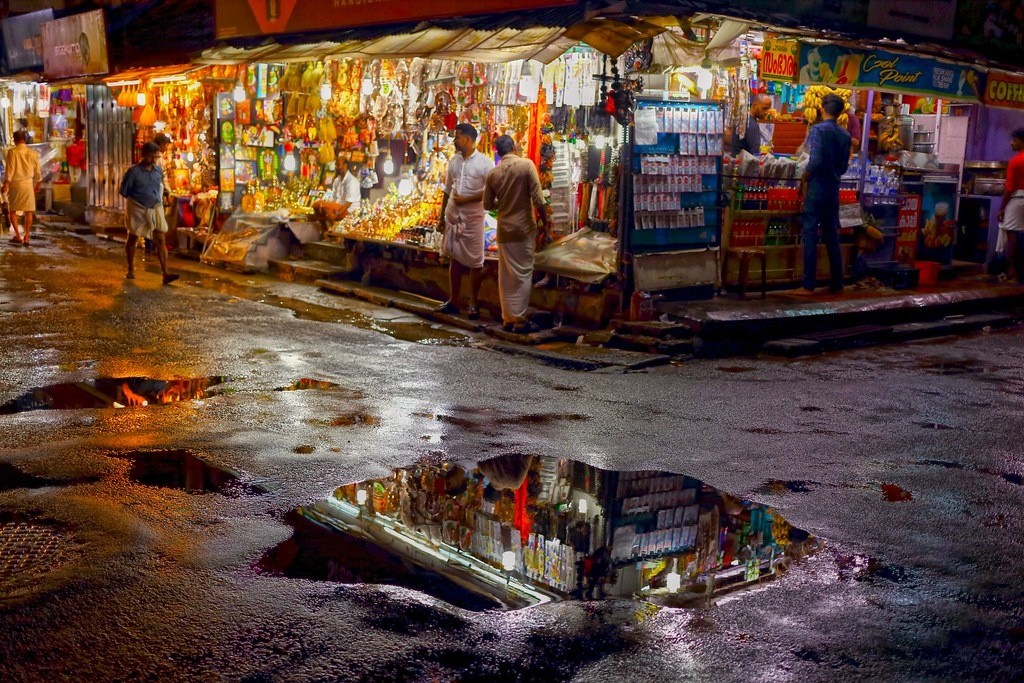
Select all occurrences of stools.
[722,247,767,302]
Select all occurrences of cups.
[832,56,859,86]
[807,50,821,81]
[936,202,949,235]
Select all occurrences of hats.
[822,94,843,114]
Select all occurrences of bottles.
[730,218,800,245]
[735,180,803,212]
[839,155,901,206]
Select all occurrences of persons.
[731,94,771,158]
[328,156,361,220]
[477,452,534,502]
[0,118,42,247]
[432,459,478,497]
[976,128,1024,285]
[484,134,549,334]
[434,123,495,320]
[784,92,852,296]
[117,134,180,284]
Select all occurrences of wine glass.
[957,74,966,95]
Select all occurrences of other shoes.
[163,274,178,284]
[21,242,29,246]
[434,301,461,314]
[503,319,540,333]
[126,273,135,278]
[468,306,480,320]
[9,237,22,243]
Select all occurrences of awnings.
[191,6,1024,78]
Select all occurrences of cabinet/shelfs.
[608,473,702,564]
[855,192,907,281]
